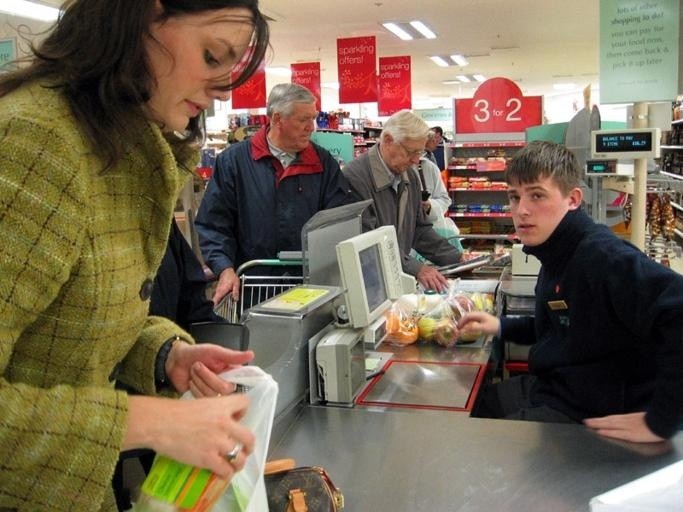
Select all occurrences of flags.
[376,56,411,117]
[597,0,678,106]
[289,62,321,112]
[335,36,378,104]
[230,46,267,110]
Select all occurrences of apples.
[434,319,460,349]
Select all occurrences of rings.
[226,443,241,464]
[217,393,221,397]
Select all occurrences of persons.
[193,82,358,308]
[335,108,481,297]
[2,2,258,509]
[417,131,436,165]
[403,148,453,249]
[111,213,233,511]
[453,139,682,446]
[427,125,451,172]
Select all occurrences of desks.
[238,286,683,512]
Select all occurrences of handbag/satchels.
[264,458,343,512]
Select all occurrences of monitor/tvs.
[338,223,407,328]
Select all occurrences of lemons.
[418,317,436,342]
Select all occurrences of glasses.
[401,142,427,157]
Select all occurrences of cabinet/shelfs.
[226,99,683,260]
